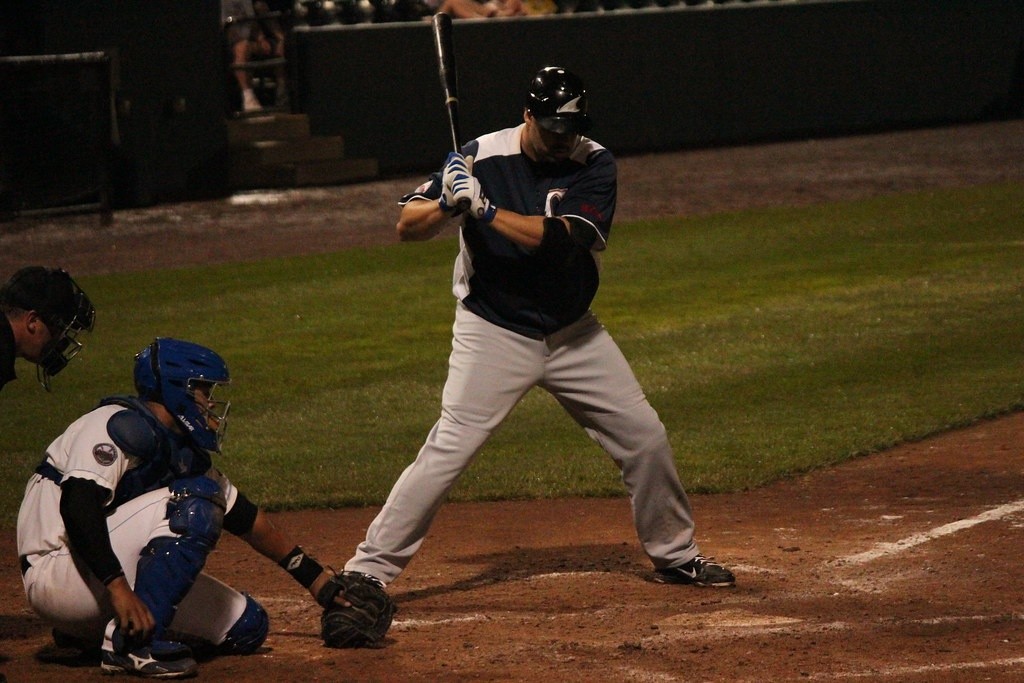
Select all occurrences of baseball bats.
[430,11,470,213]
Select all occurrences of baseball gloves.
[314,573,395,650]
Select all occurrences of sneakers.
[52,625,99,651]
[656,554,735,586]
[101,619,199,675]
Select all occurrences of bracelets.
[278,545,324,589]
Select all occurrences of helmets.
[134,339,228,451]
[8,267,95,332]
[524,61,592,134]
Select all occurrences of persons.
[17,339,350,680]
[320,67,737,589]
[0,264,96,392]
[223,0,562,111]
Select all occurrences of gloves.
[437,151,497,223]
